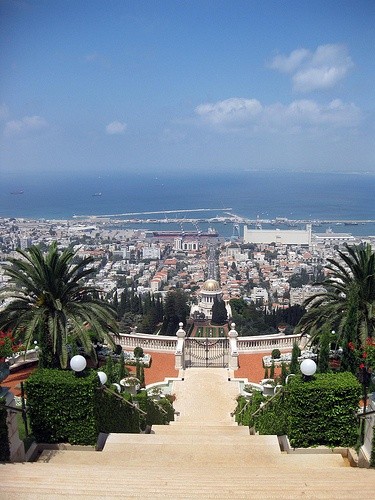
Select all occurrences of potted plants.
[91,344,153,368]
[240,378,279,401]
[262,346,343,368]
[120,377,175,403]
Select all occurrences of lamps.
[300,359,317,381]
[113,383,121,394]
[274,385,283,394]
[97,370,107,385]
[70,355,87,378]
[286,374,295,385]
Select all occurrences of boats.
[152,227,218,236]
[10,190,24,194]
[92,193,102,196]
